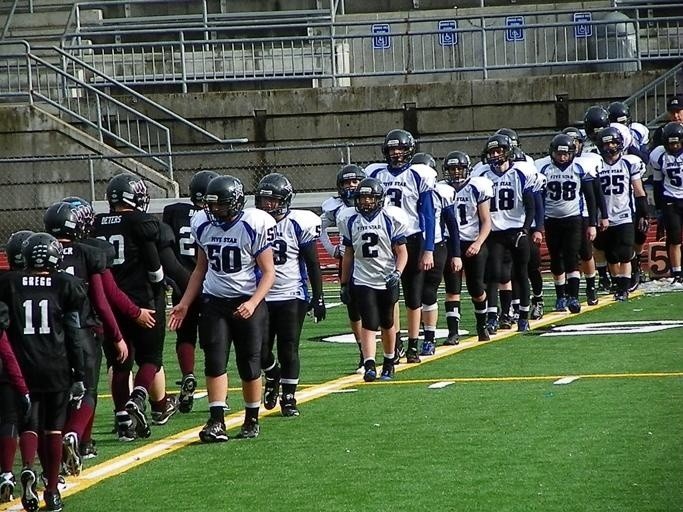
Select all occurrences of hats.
[667,95,683,110]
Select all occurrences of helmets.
[482,127,518,162]
[6,230,63,269]
[189,170,247,226]
[42,196,95,240]
[355,177,385,211]
[662,123,683,153]
[336,164,367,205]
[584,101,631,126]
[254,172,294,217]
[106,172,150,213]
[550,126,584,164]
[441,150,472,182]
[597,127,624,155]
[381,128,437,168]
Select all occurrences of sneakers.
[176,375,197,413]
[199,420,228,441]
[43,490,62,512]
[357,274,641,382]
[118,420,134,442]
[264,383,279,410]
[0,472,15,502]
[241,418,259,437]
[82,441,96,458]
[125,400,151,438]
[61,431,81,478]
[280,399,300,416]
[19,467,40,512]
[150,395,177,424]
